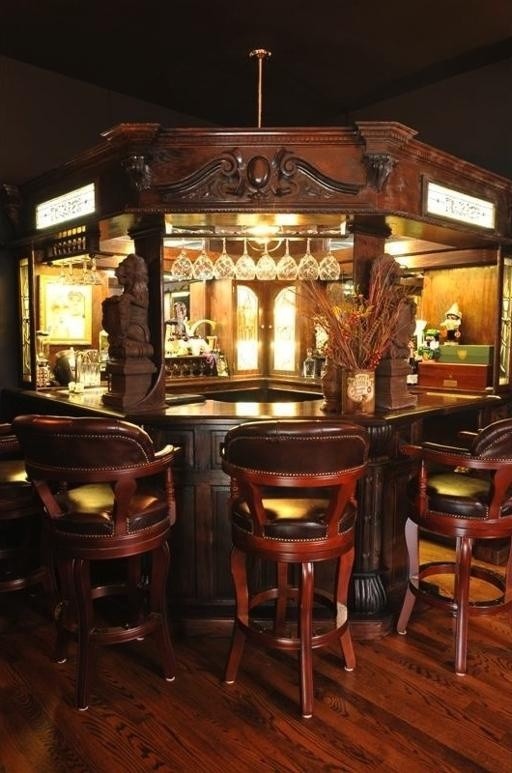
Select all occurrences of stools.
[2,422,70,664]
[217,419,369,718]
[15,415,182,714]
[396,417,512,675]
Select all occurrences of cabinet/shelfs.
[213,278,316,379]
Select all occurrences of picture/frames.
[38,274,92,346]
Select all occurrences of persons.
[440,303,463,342]
[407,336,417,373]
[417,327,439,361]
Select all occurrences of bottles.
[303,347,315,379]
